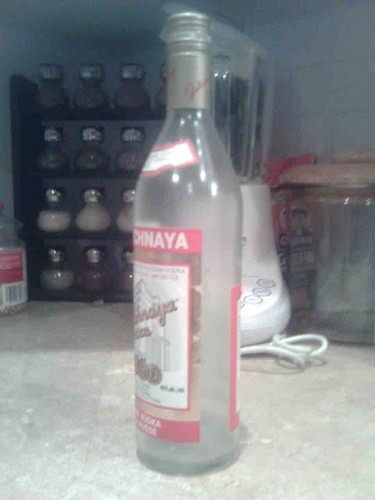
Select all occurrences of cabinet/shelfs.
[9,66,245,305]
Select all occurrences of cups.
[222,175,291,346]
[307,180,375,341]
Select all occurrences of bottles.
[39,49,152,292]
[0,203,31,316]
[133,11,249,479]
